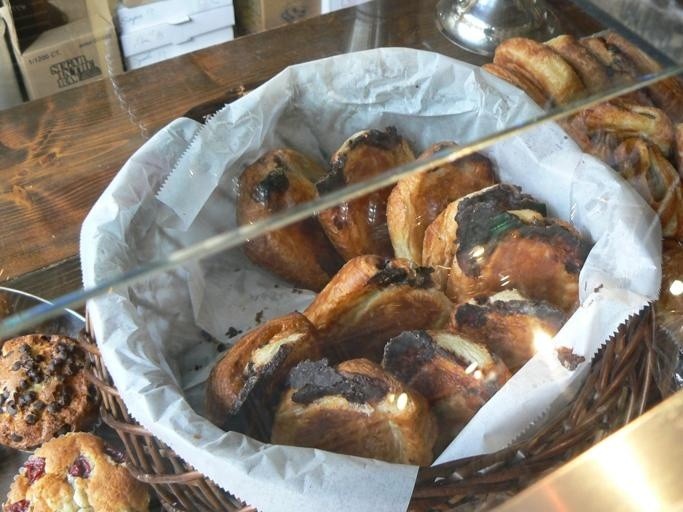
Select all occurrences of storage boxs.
[236,0,322,37]
[0,17,27,111]
[0,0,125,102]
[114,0,236,71]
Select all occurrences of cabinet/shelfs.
[0,0,682,512]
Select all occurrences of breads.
[649,73,683,123]
[608,32,656,72]
[613,91,659,112]
[314,131,414,255]
[482,61,553,110]
[544,35,612,94]
[566,112,683,239]
[0,333,102,453]
[426,184,543,291]
[207,310,317,439]
[583,37,635,86]
[455,291,570,372]
[306,254,451,365]
[446,208,589,320]
[672,123,683,179]
[586,99,674,151]
[268,358,436,469]
[237,147,341,290]
[381,327,514,441]
[493,37,590,108]
[387,139,500,271]
[3,435,150,511]
